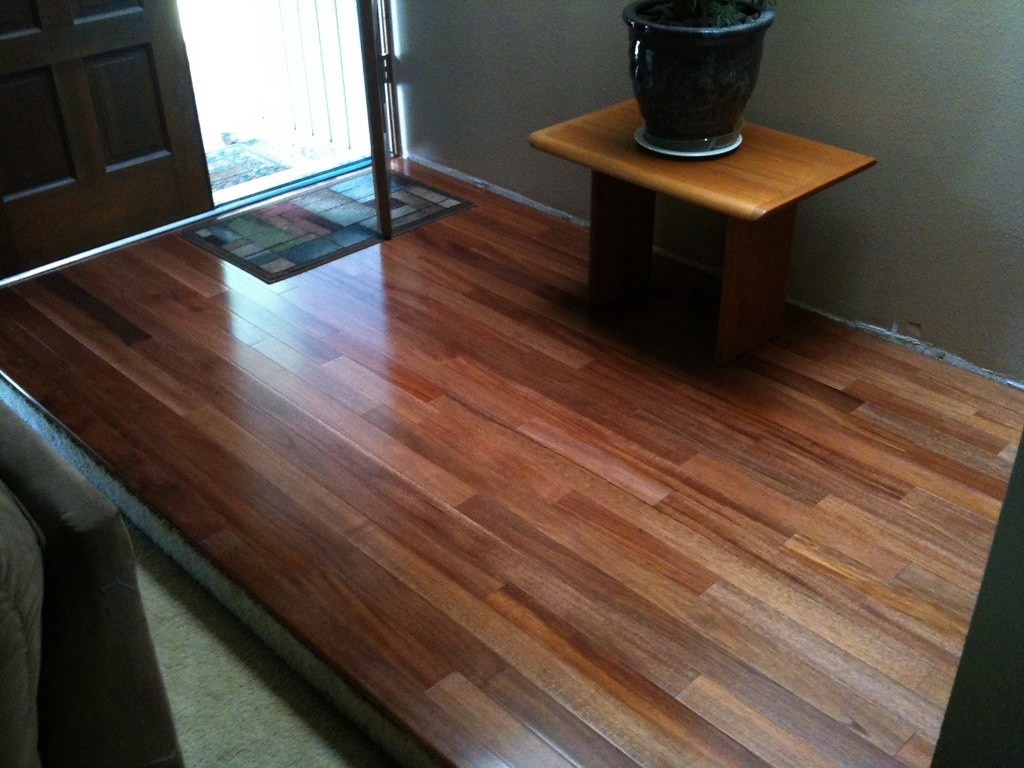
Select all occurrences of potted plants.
[623,0,776,157]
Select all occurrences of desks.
[527,97,878,365]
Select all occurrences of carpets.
[179,168,479,285]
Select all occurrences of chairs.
[0,401,187,768]
